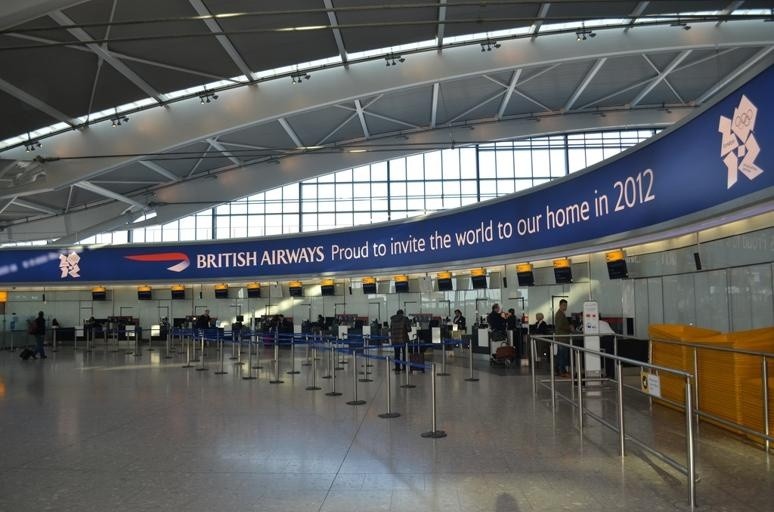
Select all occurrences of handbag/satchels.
[495,346,513,359]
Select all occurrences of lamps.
[22,130,43,153]
[289,67,311,86]
[576,23,596,42]
[670,13,692,32]
[110,107,129,128]
[479,33,501,53]
[383,52,406,66]
[197,85,219,104]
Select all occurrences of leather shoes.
[41,355,47,358]
[560,372,569,377]
[32,354,37,359]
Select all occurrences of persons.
[278,314,290,334]
[332,320,339,337]
[197,310,215,329]
[453,309,465,331]
[31,311,47,359]
[318,314,325,330]
[486,299,583,378]
[390,310,411,372]
[10,313,18,329]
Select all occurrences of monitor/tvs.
[138,290,152,301]
[437,278,453,291]
[321,285,334,296]
[171,290,185,300]
[516,271,534,287]
[288,287,304,297]
[554,267,572,285]
[605,260,629,279]
[248,288,260,299]
[363,283,376,294]
[92,292,107,302]
[471,275,487,290]
[215,289,228,299]
[395,281,409,293]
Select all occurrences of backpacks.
[28,320,40,335]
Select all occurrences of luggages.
[19,349,33,360]
[410,352,425,371]
